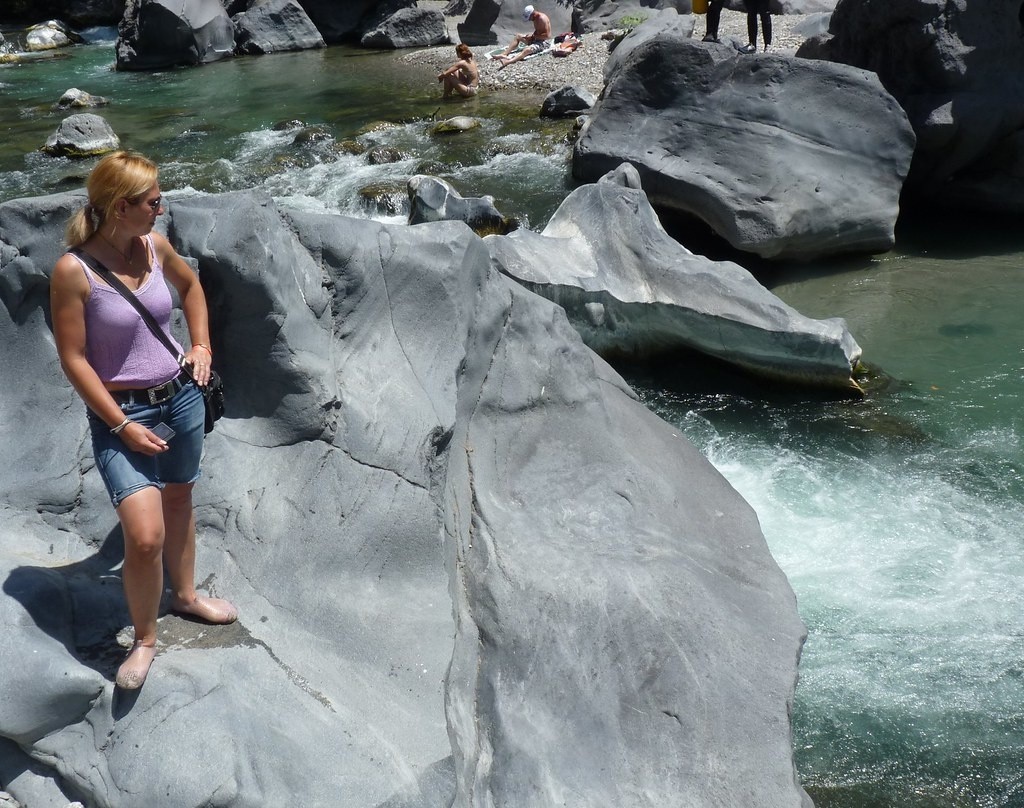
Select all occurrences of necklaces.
[99,230,134,265]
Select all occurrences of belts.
[112,368,191,406]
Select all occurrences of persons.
[702,0,725,42]
[492,5,551,66]
[437,43,478,97]
[739,0,775,53]
[51,152,238,690]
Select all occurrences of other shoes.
[180,593,238,624]
[116,642,157,689]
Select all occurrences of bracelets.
[110,418,130,436]
[192,344,212,355]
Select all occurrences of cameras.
[149,422,176,443]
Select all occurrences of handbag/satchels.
[201,370,225,434]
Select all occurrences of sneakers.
[763,43,772,54]
[739,43,756,54]
[702,33,720,43]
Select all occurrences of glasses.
[135,193,162,210]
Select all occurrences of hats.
[522,5,534,22]
